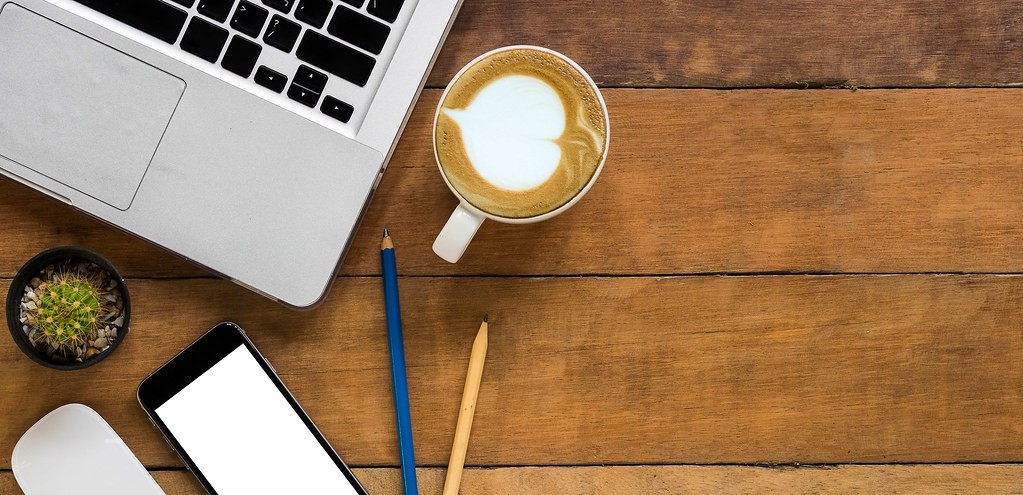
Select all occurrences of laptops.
[0,0,466,311]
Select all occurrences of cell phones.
[137,321,370,495]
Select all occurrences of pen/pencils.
[379,229,418,495]
[443,314,489,495]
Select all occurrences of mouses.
[12,403,166,495]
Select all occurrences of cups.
[432,44,610,264]
